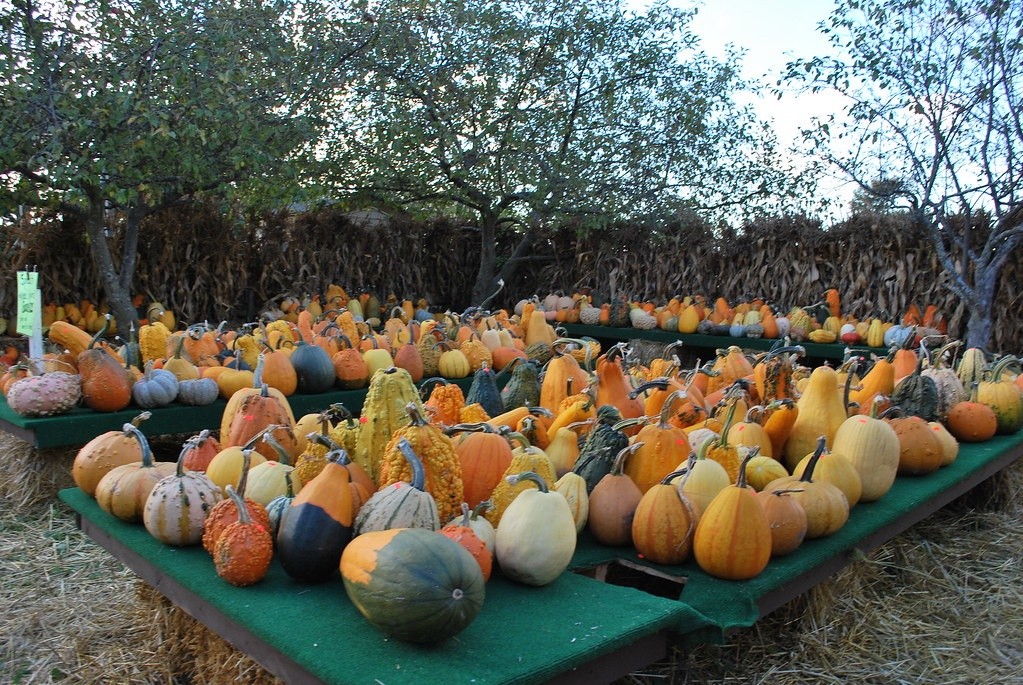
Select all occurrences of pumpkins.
[0,284,1023,645]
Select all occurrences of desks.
[0,321,1023,685]
